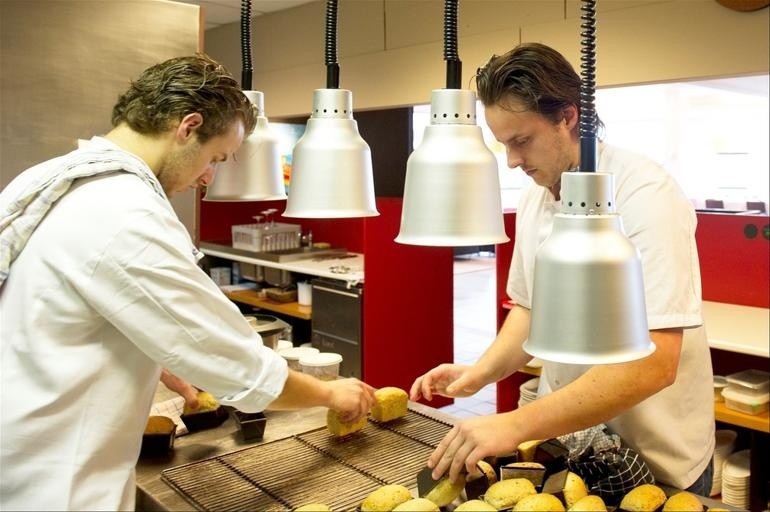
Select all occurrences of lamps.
[391,0,513,247]
[286,1,383,218]
[520,0,656,364]
[196,2,285,203]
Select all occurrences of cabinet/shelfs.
[503,266,770,438]
[197,249,365,380]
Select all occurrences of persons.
[0,49,379,512]
[410,43,716,497]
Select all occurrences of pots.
[243,313,294,350]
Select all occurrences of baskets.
[232,223,302,253]
[266,287,297,303]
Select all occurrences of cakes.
[417,468,447,498]
[542,471,588,509]
[477,460,499,488]
[512,493,565,512]
[518,439,546,461]
[423,475,467,508]
[533,438,570,476]
[369,387,409,422]
[565,494,608,512]
[183,392,221,415]
[499,462,545,487]
[326,408,368,437]
[390,497,441,512]
[620,484,667,511]
[465,476,489,501]
[453,499,497,512]
[292,502,332,512]
[484,451,519,477]
[232,409,268,442]
[360,485,412,512]
[483,477,538,512]
[662,492,704,512]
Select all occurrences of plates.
[721,450,750,509]
[707,429,737,497]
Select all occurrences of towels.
[0,145,204,288]
